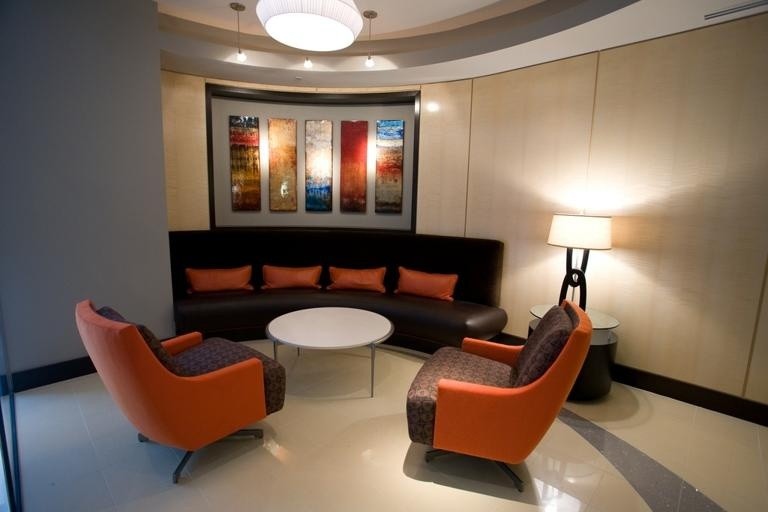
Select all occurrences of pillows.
[185,266,254,291]
[326,265,388,293]
[261,264,323,290]
[394,266,459,304]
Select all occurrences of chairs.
[406,301,593,492]
[76,300,286,485]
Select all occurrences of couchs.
[170,229,508,350]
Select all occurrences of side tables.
[528,305,620,401]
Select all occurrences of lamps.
[304,56,312,69]
[235,9,246,61]
[547,212,612,312]
[365,16,376,69]
[255,0,363,51]
